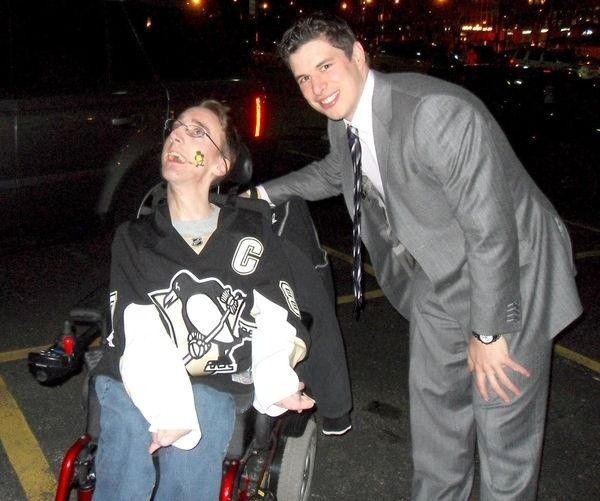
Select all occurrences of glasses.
[162,119,227,158]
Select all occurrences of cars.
[0,1,269,250]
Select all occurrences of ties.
[350,124,363,314]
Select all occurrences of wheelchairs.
[27,182,317,501]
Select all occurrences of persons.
[75,98,317,501]
[224,10,584,500]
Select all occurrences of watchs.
[472,331,500,345]
